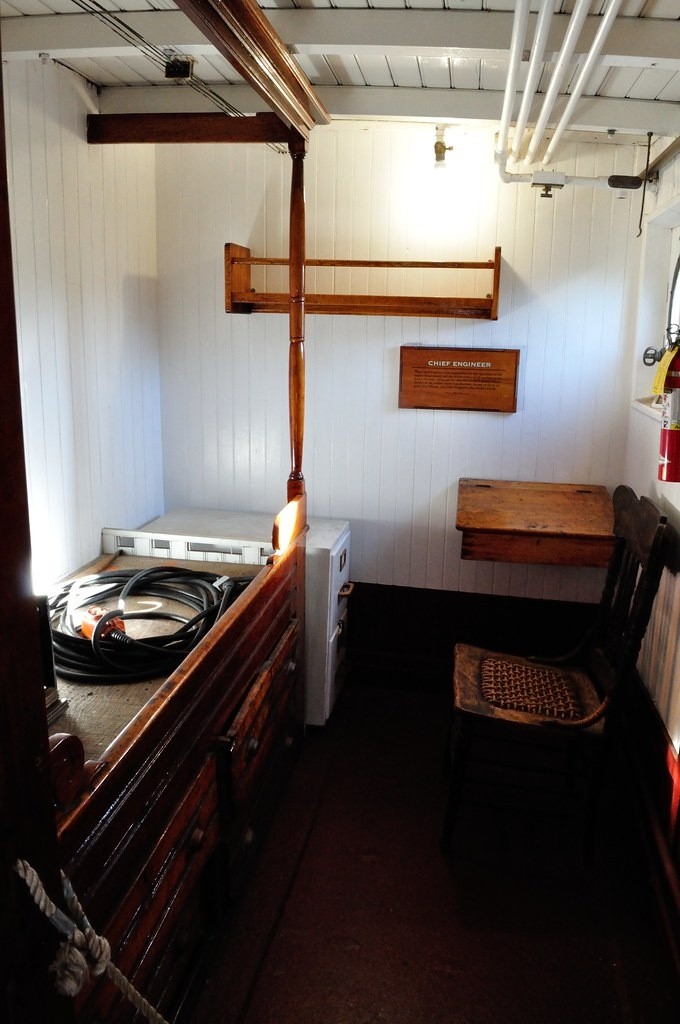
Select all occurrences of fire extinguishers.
[657,323,680,483]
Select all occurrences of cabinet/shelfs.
[138,509,353,726]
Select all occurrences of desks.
[455,478,617,569]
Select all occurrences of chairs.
[443,484,668,891]
